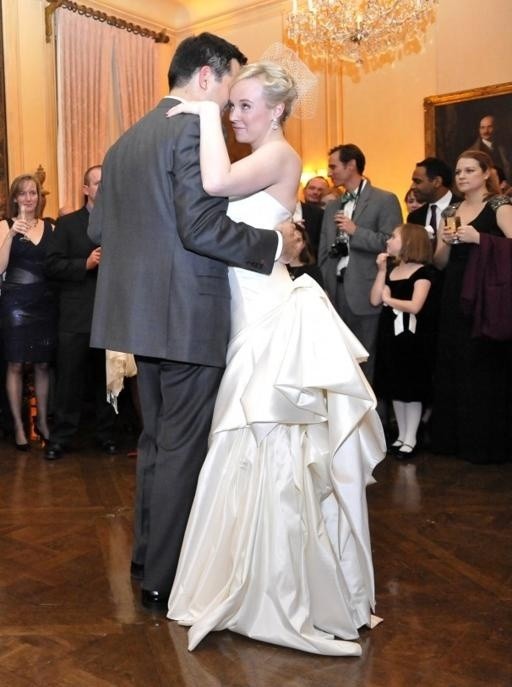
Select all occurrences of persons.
[40,165,128,461]
[0,174,57,451]
[163,60,388,658]
[86,31,303,609]
[369,115,512,460]
[292,143,404,389]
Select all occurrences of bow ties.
[340,189,356,203]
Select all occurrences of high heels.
[34,425,51,443]
[16,435,31,452]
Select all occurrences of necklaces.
[15,217,39,235]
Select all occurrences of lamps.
[283,0,440,84]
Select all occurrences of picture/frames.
[423,82,512,201]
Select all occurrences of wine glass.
[19,206,31,242]
[333,212,349,246]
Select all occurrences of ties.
[430,205,437,253]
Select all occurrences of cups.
[444,204,461,245]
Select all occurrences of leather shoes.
[140,589,175,621]
[103,440,118,453]
[387,440,403,455]
[396,441,420,459]
[44,441,62,460]
[130,560,145,579]
